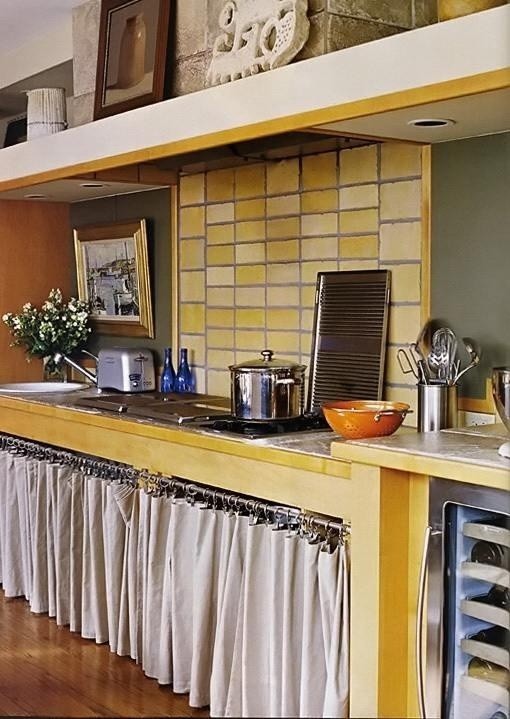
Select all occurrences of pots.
[229,349,307,425]
[320,399,414,442]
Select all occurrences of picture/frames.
[91,2,176,121]
[73,216,157,341]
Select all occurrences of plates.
[1,382,89,394]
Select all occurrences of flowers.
[2,286,93,382]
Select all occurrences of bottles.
[160,348,175,392]
[176,348,192,393]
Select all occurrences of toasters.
[97,349,156,395]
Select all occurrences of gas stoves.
[199,413,344,441]
